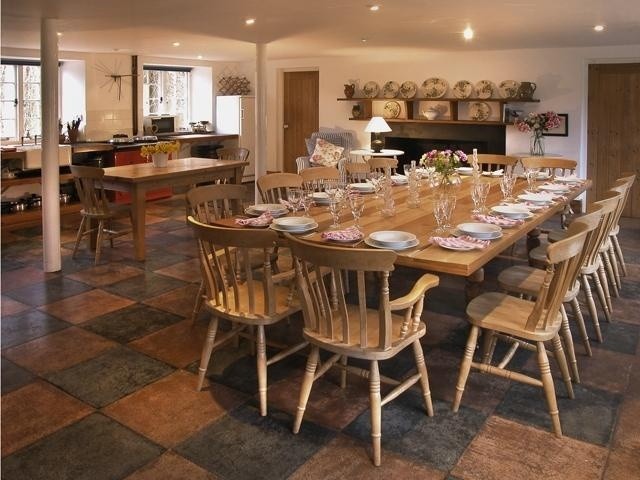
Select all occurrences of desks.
[87,157,250,262]
[212,176,593,322]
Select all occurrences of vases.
[152,153,169,168]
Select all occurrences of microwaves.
[144,113,182,137]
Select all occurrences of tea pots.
[421,106,440,120]
[518,81,537,99]
[343,83,356,98]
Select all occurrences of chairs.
[186,148,249,225]
[283,230,439,467]
[188,214,323,417]
[257,157,400,275]
[480,169,636,384]
[69,165,133,266]
[452,222,589,440]
[467,154,578,179]
[185,184,249,327]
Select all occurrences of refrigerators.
[214,94,256,184]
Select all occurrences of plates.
[383,101,401,119]
[369,231,417,247]
[364,237,420,251]
[269,222,319,234]
[361,79,518,99]
[272,217,316,229]
[250,204,286,214]
[470,103,490,120]
[244,208,290,219]
[439,242,478,251]
[287,164,584,242]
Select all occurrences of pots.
[9,191,72,213]
[106,135,158,146]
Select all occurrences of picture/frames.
[535,113,569,137]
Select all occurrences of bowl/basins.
[59,135,66,144]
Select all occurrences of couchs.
[294,126,354,183]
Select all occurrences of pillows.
[309,137,344,169]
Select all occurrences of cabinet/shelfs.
[335,98,542,126]
[2,144,113,231]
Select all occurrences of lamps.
[364,116,393,153]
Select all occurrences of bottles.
[352,105,362,118]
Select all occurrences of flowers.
[424,147,468,191]
[139,140,180,158]
[512,110,562,147]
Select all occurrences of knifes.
[66,118,82,129]
